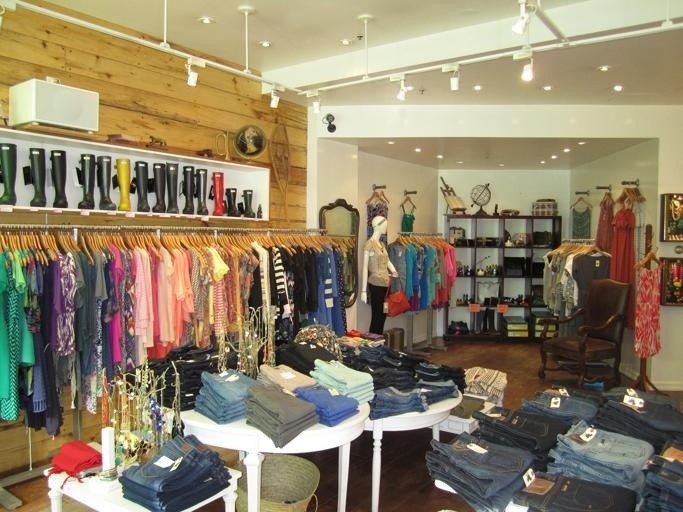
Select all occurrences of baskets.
[231,454,320,512]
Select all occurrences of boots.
[0,144,255,218]
[484,298,490,306]
[473,308,496,334]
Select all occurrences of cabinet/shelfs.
[444,215,562,342]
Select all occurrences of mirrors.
[319,199,360,307]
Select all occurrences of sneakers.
[457,298,464,305]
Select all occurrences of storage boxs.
[503,311,557,337]
[532,202,558,216]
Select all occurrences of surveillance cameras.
[326,113,334,123]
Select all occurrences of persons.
[360,215,399,335]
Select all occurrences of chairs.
[538,279,630,390]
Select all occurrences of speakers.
[8,78,99,134]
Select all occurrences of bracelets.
[361,291,368,292]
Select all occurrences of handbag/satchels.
[384,289,411,317]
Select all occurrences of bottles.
[485,264,497,277]
[456,294,469,306]
[462,265,474,275]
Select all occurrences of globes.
[470,183,491,216]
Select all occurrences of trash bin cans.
[389,327,404,351]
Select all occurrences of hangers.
[365,185,386,204]
[400,192,417,209]
[375,185,390,204]
[388,231,449,252]
[570,191,593,209]
[634,245,664,270]
[547,238,613,260]
[331,234,356,250]
[599,180,647,206]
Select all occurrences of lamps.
[522,57,533,81]
[511,0,529,34]
[451,65,460,92]
[313,92,323,115]
[271,82,280,110]
[397,74,408,101]
[187,57,198,87]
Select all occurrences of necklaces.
[217,303,276,378]
[103,358,181,466]
